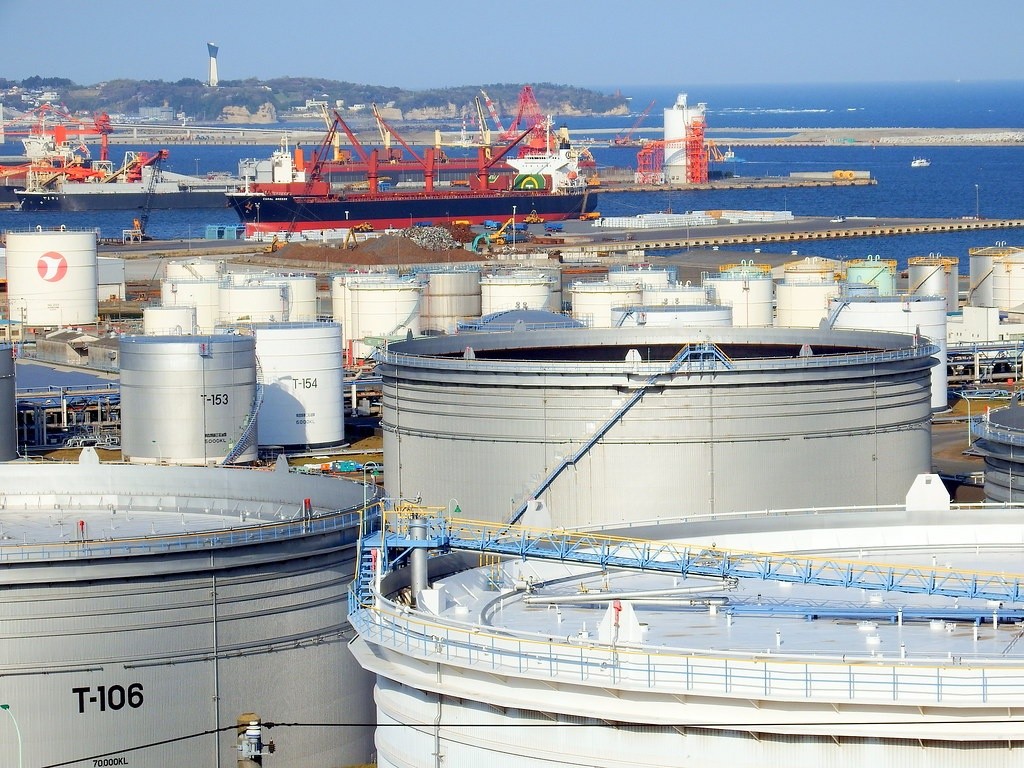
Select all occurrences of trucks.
[451,220,470,225]
[484,209,564,245]
[580,212,600,221]
[414,221,432,227]
[351,222,374,232]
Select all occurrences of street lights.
[446,210,450,265]
[410,213,412,226]
[975,184,979,217]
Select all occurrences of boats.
[0,103,330,212]
[707,139,745,164]
[911,156,931,167]
[225,109,600,235]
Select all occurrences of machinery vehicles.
[263,237,290,252]
[127,151,165,241]
[340,229,359,249]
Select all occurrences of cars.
[830,214,846,224]
